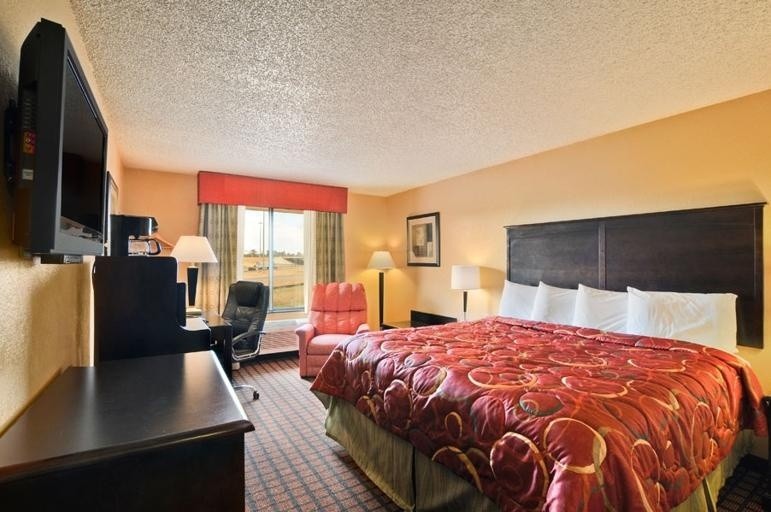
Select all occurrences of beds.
[308,202,765,512]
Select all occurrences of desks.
[200,310,233,384]
[0,348,254,511]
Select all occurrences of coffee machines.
[110,213,162,257]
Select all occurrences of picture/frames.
[406,210,440,267]
[104,170,119,256]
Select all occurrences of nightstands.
[762,396,771,461]
[381,319,444,328]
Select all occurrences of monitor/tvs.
[14,18,107,266]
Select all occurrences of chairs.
[218,280,270,399]
[294,281,371,378]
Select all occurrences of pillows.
[530,281,578,327]
[573,283,629,335]
[497,279,539,320]
[626,285,739,354]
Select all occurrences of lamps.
[366,250,393,330]
[169,235,218,315]
[451,265,480,320]
